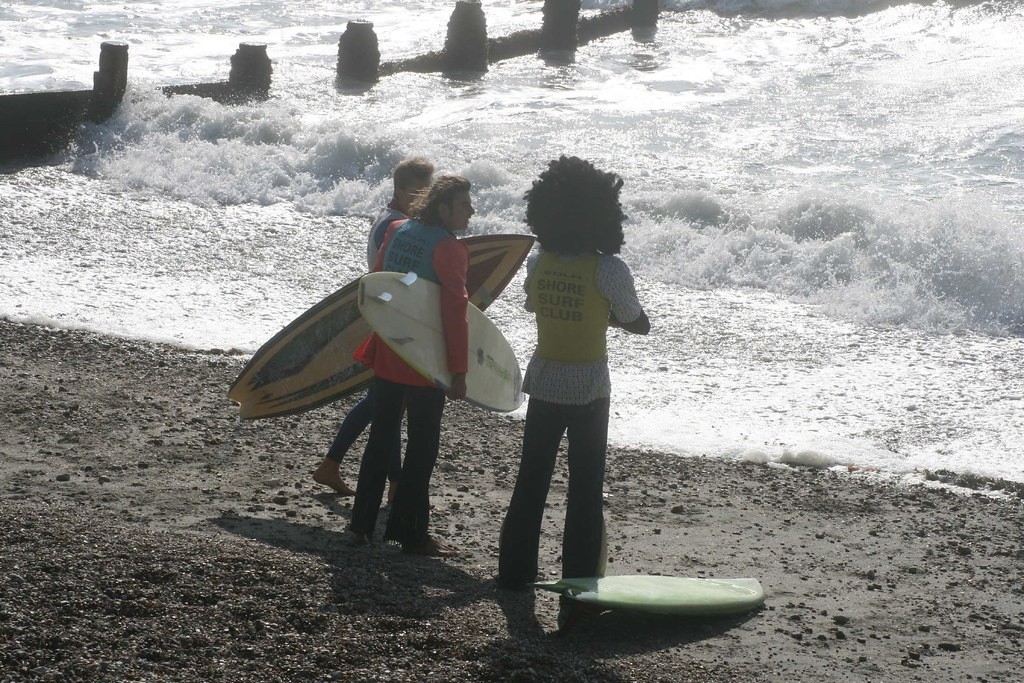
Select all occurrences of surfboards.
[529,575,764,617]
[226,234,536,421]
[357,271,524,413]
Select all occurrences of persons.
[498,155,651,591]
[348,175,476,559]
[314,158,438,512]
